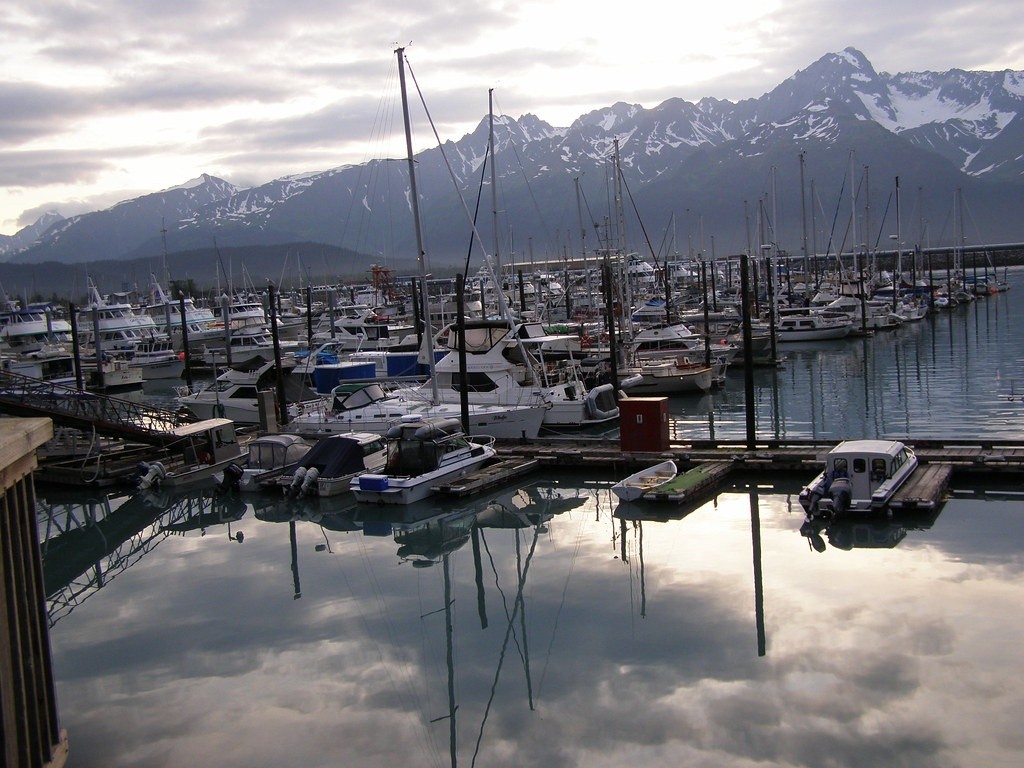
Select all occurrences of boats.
[210,435,314,494]
[611,459,678,503]
[801,439,919,521]
[133,419,260,489]
[347,413,497,507]
[0,42,1012,442]
[277,433,393,499]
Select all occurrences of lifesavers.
[581,336,592,348]
